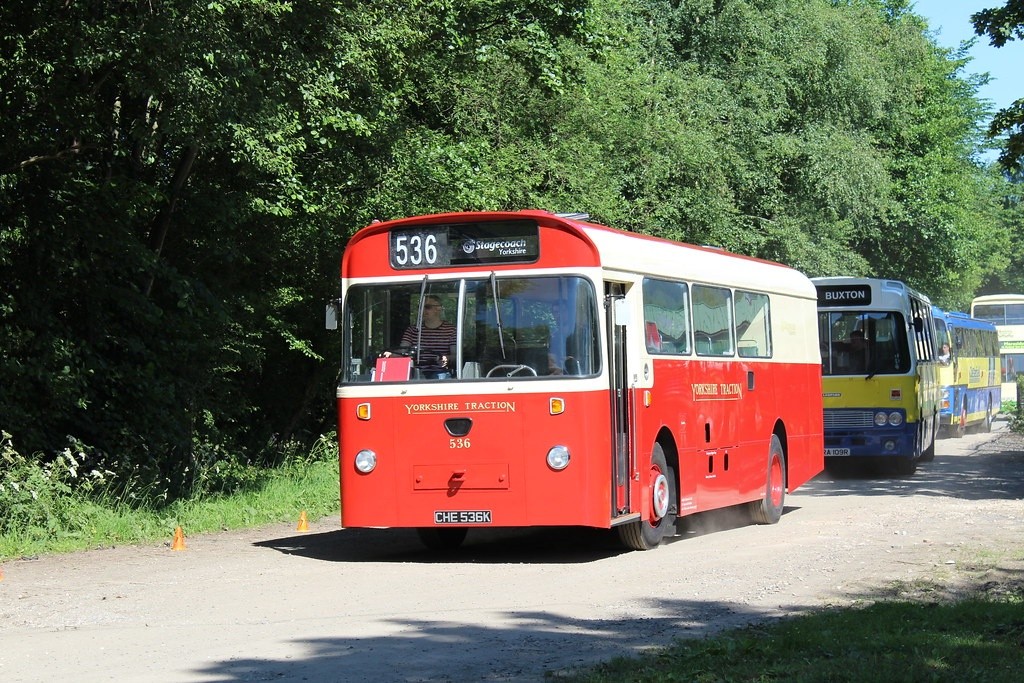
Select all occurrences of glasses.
[418,304,441,310]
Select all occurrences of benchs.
[660,328,713,355]
[738,339,758,356]
[484,339,550,375]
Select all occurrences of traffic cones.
[294,511,310,533]
[169,526,188,552]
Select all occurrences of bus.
[797,273,943,478]
[319,208,826,549]
[969,294,1024,406]
[930,304,1004,439]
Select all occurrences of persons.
[848,330,871,371]
[938,343,950,363]
[379,294,457,380]
[547,298,596,376]
